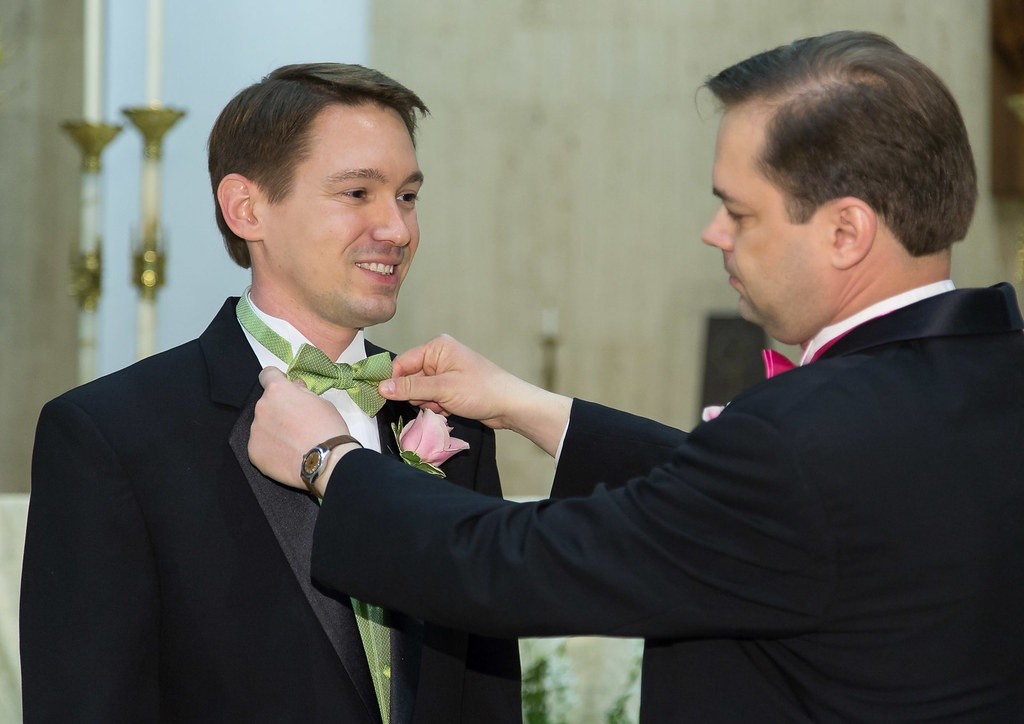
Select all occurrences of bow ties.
[761,326,859,379]
[235,287,392,419]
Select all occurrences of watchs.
[299,435,364,500]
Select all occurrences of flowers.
[390,408,470,479]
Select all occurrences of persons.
[19,60,523,723]
[247,27,1024,724]
